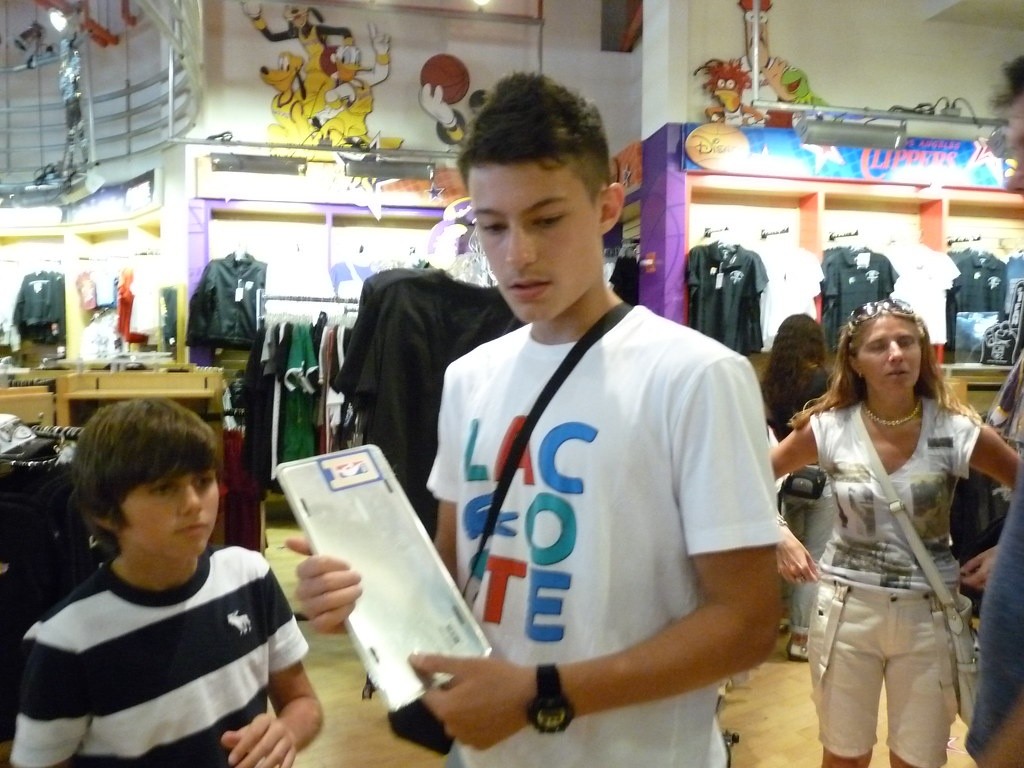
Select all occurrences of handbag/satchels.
[948,593,980,729]
[387,701,454,756]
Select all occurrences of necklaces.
[861,396,921,427]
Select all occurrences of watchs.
[530,663,574,734]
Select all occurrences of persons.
[758,312,837,660]
[773,299,1024,768]
[8,396,325,768]
[285,73,787,768]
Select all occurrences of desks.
[0,368,227,549]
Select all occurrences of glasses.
[847,299,917,325]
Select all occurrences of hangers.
[827,232,873,261]
[699,227,738,257]
[31,425,87,441]
[761,228,800,253]
[948,236,994,263]
[260,295,360,330]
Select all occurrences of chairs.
[978,281,1024,365]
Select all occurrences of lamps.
[795,113,906,151]
[901,117,1008,149]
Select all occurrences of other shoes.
[786,643,811,662]
